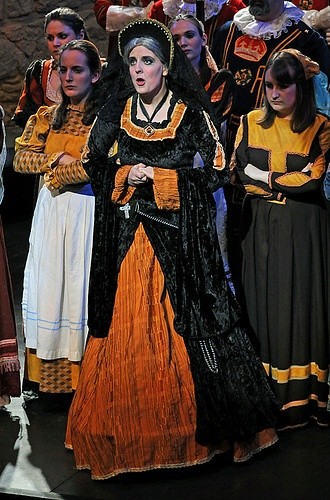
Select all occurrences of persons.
[0,0,330,431]
[64,18,282,480]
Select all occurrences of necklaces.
[139,89,170,136]
